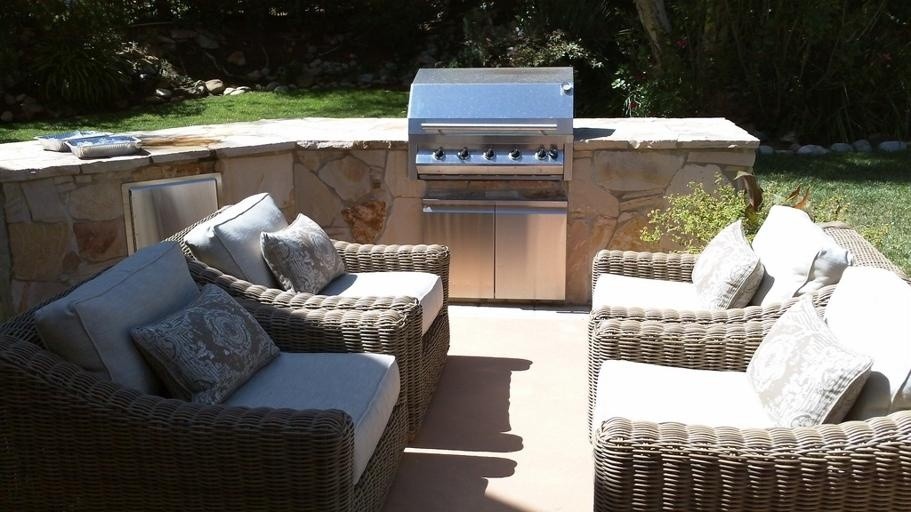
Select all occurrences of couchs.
[586,289,836,511]
[582,200,836,440]
[1,241,419,510]
[161,191,453,445]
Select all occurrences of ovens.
[408,66,574,182]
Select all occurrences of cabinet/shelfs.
[420,196,570,304]
